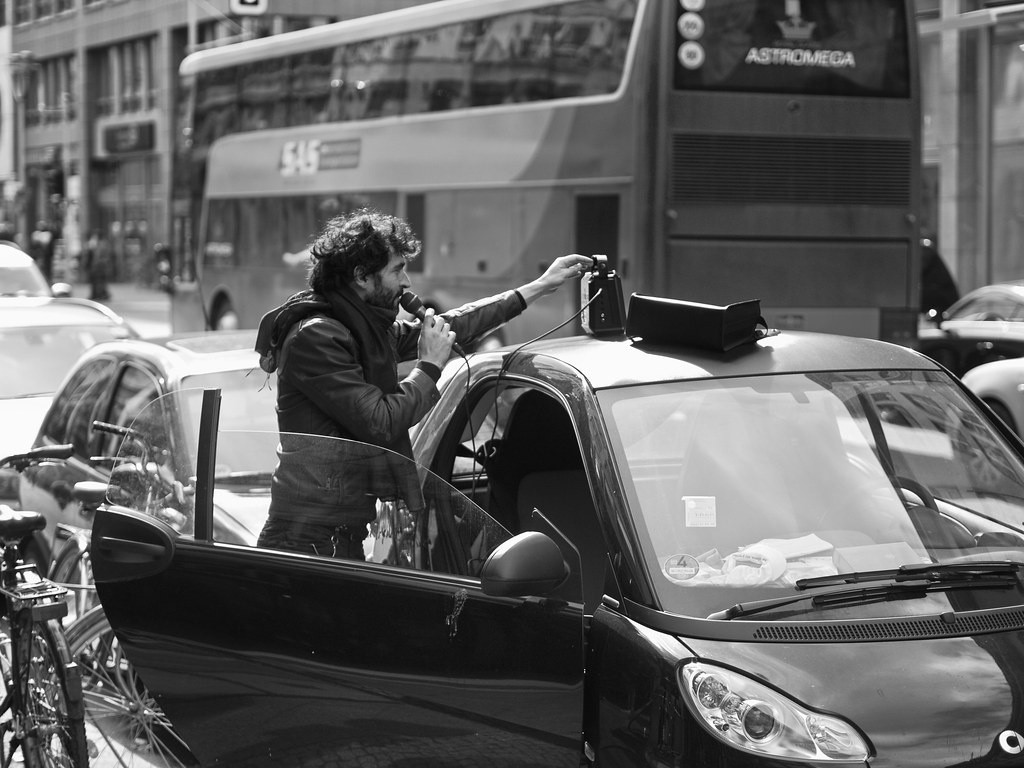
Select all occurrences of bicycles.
[44,417,210,768]
[1,443,93,768]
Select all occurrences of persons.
[254,212,594,561]
[0,210,110,301]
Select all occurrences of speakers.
[579,254,628,337]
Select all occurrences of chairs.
[482,387,612,612]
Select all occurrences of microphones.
[399,291,466,359]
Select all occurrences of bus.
[178,0,925,354]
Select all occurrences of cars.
[918,278,1024,379]
[16,333,378,688]
[0,291,147,506]
[91,324,1024,768]
